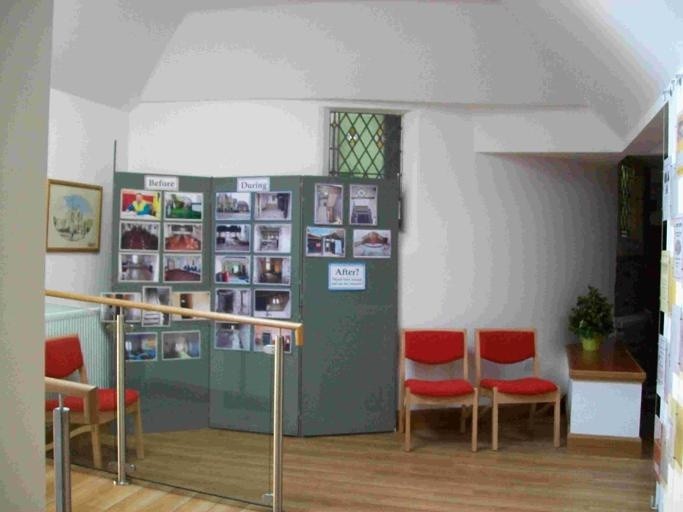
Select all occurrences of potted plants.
[568,283,614,352]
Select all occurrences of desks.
[564,339,647,458]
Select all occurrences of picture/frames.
[46,178,104,254]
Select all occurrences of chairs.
[474,327,561,451]
[44,333,145,470]
[398,328,480,453]
